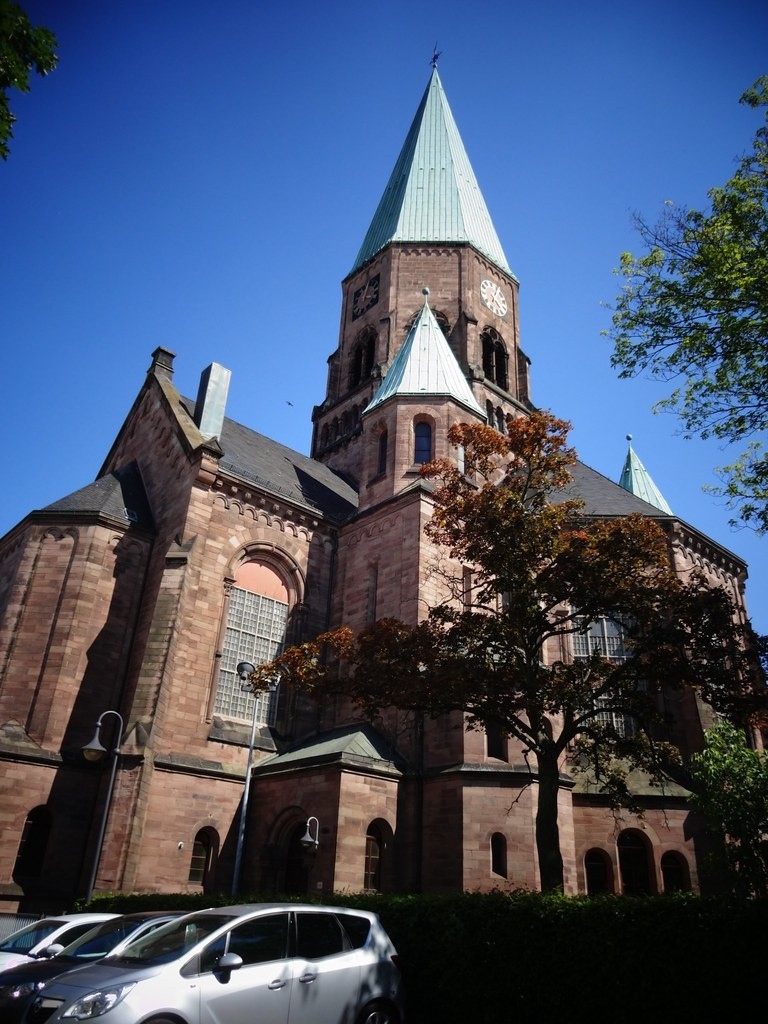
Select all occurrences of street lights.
[301,816,320,903]
[81,710,124,903]
[230,663,292,902]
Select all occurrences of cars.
[0,909,271,1024]
[0,912,157,974]
[24,903,399,1024]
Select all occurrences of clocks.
[480,280,508,317]
[352,273,380,323]
